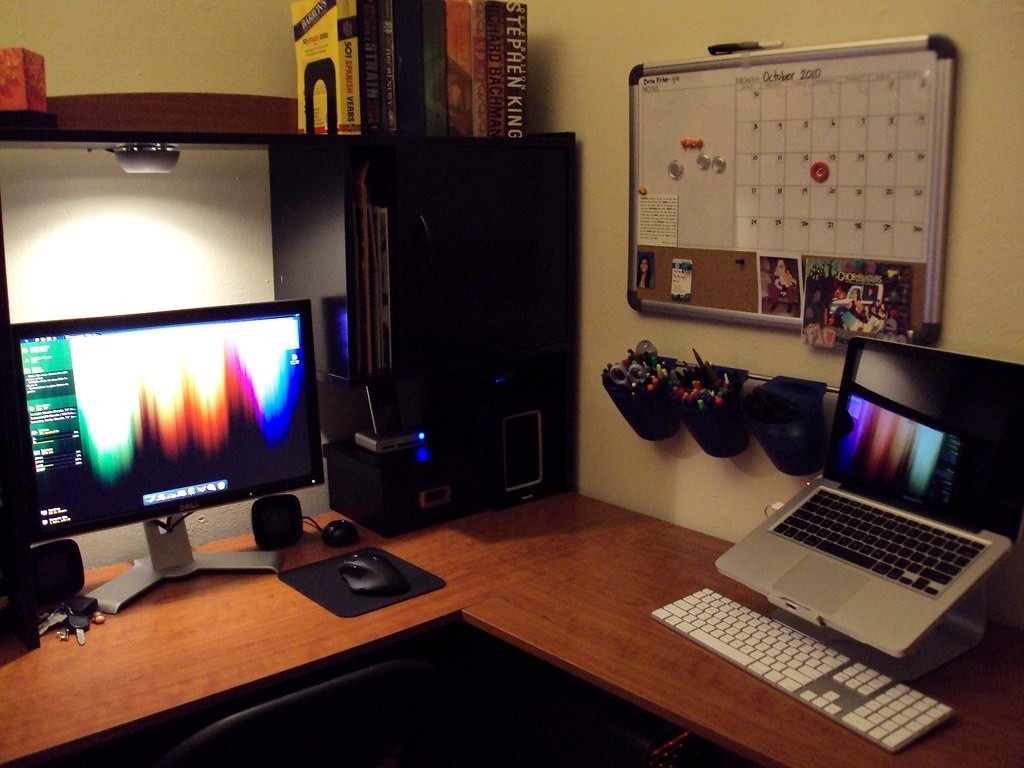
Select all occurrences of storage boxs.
[0,47,46,112]
[291,0,526,138]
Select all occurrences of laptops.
[717,336,1024,655]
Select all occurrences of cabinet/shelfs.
[273,135,580,510]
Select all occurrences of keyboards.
[648,588,957,753]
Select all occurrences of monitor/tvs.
[7,295,328,613]
[838,382,970,523]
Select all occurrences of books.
[291,0,527,138]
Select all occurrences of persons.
[637,254,655,288]
[767,259,797,315]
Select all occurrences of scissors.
[609,364,646,390]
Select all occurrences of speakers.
[31,541,84,612]
[249,494,303,549]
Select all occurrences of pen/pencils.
[601,348,732,410]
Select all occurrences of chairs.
[168,653,415,768]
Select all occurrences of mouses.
[338,551,408,596]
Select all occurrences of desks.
[0,508,1024,768]
